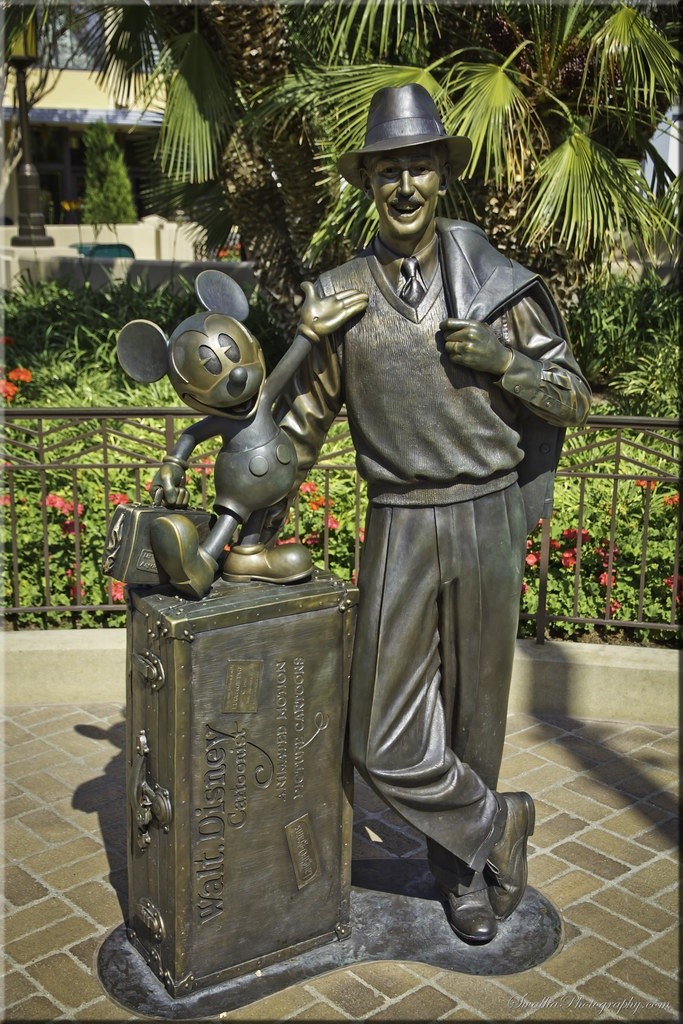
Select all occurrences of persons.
[271,83,591,944]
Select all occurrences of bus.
[0,57,315,260]
